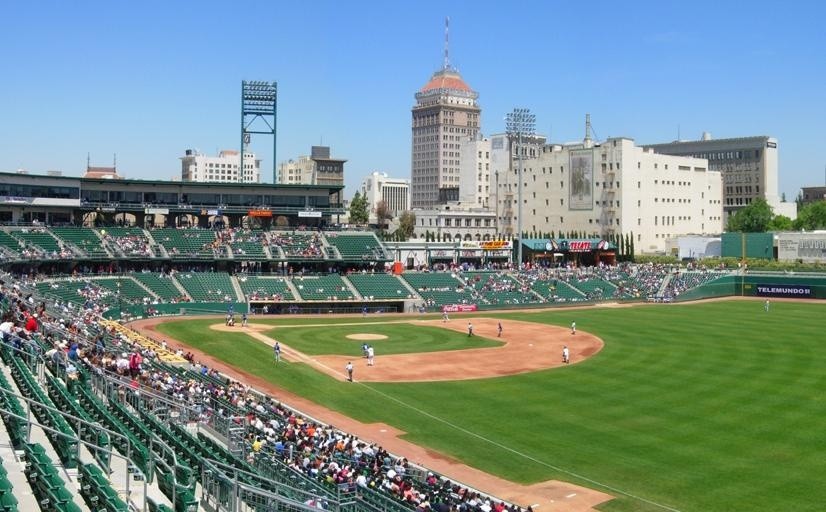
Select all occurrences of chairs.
[1,227,729,512]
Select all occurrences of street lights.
[503,106,539,272]
[239,78,277,186]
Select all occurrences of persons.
[0,227,323,325]
[571,320,576,335]
[468,322,475,337]
[0,281,298,439]
[498,322,502,337]
[442,310,449,323]
[245,415,533,512]
[563,345,569,363]
[764,299,769,312]
[327,260,748,306]
[346,343,379,382]
